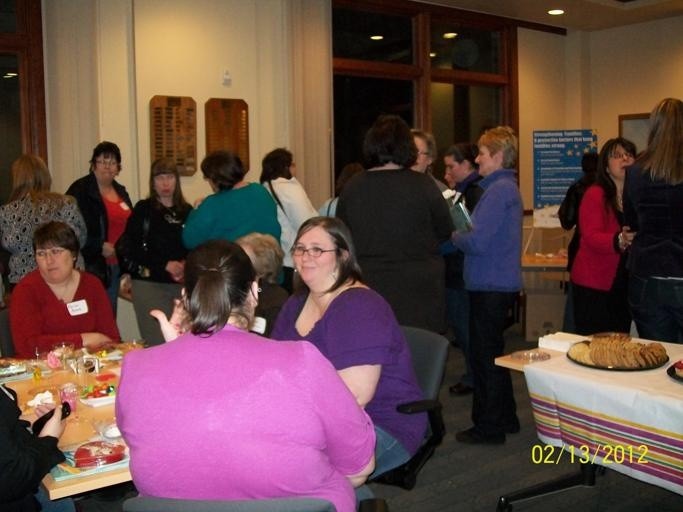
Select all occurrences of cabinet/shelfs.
[521,214,576,349]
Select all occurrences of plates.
[80,395,119,407]
[103,423,123,440]
[567,339,683,383]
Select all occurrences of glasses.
[291,248,336,257]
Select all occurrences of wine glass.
[53,342,73,373]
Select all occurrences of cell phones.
[31,401,71,432]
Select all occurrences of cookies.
[568,332,667,369]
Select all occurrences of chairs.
[115,491,389,512]
[365,324,449,488]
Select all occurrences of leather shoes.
[449,382,474,395]
[455,415,520,444]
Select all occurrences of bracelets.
[616,232,621,249]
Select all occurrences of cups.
[59,388,78,411]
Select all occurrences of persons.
[0,141,319,356]
[317,116,524,443]
[1,386,76,512]
[269,215,429,505]
[570,137,641,334]
[621,98,682,343]
[559,153,604,330]
[116,238,376,511]
